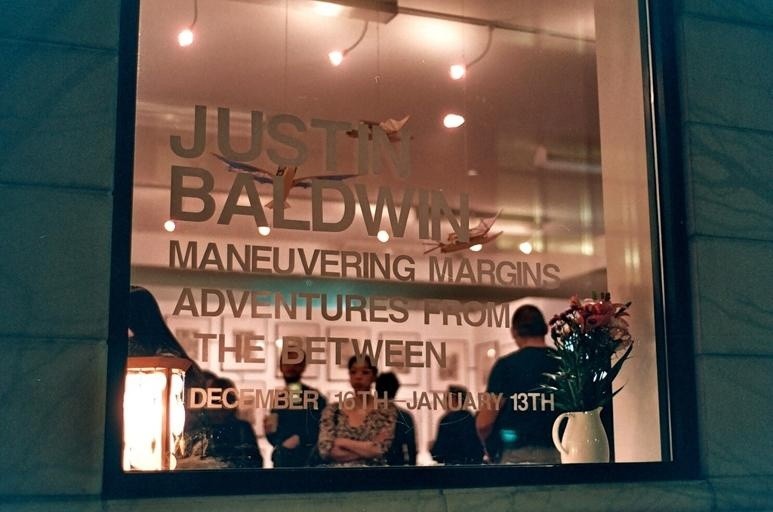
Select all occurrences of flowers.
[539,290,633,408]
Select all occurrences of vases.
[552,406,609,464]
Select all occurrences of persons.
[316,353,417,470]
[475,304,567,464]
[426,386,484,465]
[184,362,265,470]
[264,344,325,468]
